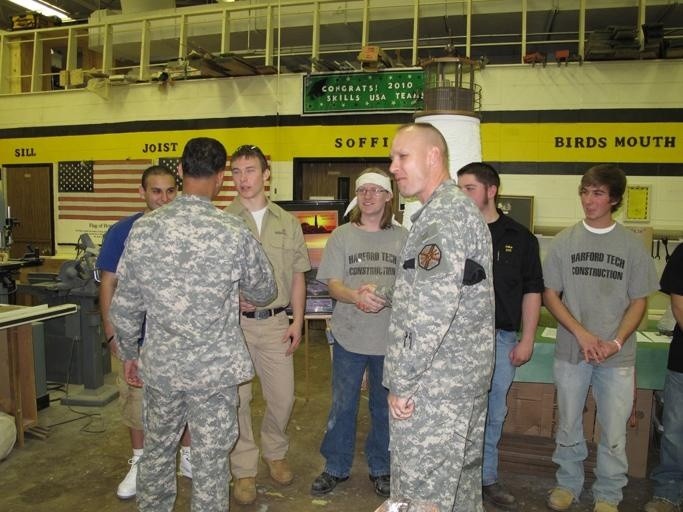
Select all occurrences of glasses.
[354,187,390,196]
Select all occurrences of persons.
[107,138,276,511]
[312,167,392,500]
[372,122,494,512]
[94,165,193,501]
[540,165,660,511]
[457,163,546,510]
[216,142,312,504]
[643,241,682,511]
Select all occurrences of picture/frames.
[625,186,652,224]
[268,195,352,320]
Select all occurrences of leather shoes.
[483,482,519,511]
[642,496,676,512]
[232,474,259,506]
[262,456,295,487]
[591,500,620,511]
[546,485,575,511]
[368,472,391,498]
[309,469,351,496]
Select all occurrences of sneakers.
[116,453,146,501]
[178,448,194,480]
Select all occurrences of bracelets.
[106,335,113,343]
[613,339,621,352]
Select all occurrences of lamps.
[355,43,395,76]
[149,67,176,85]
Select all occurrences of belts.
[239,306,288,321]
